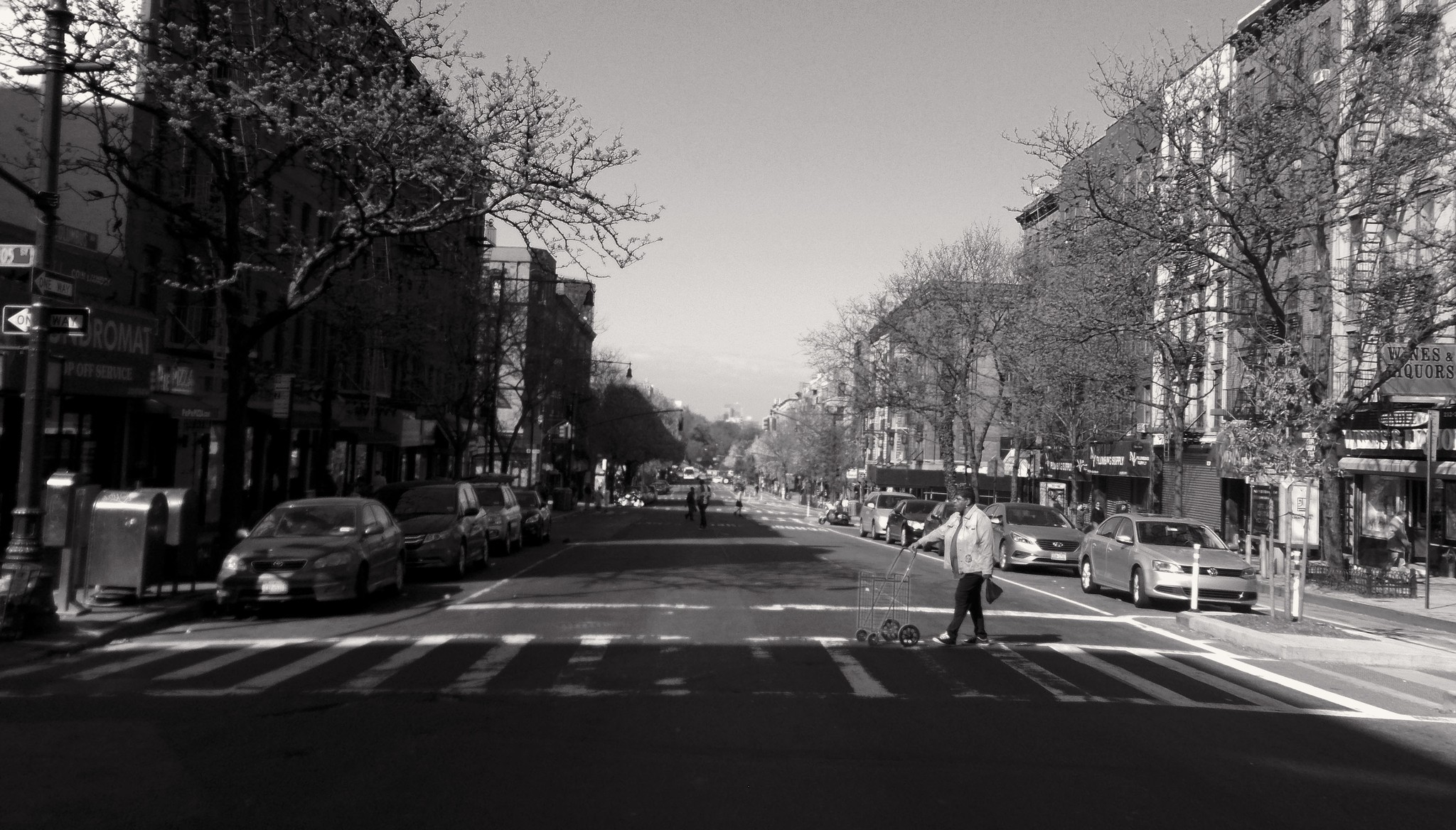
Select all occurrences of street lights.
[566,357,633,489]
[489,266,595,473]
[0,1,108,635]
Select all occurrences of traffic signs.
[31,265,76,306]
[1,302,92,338]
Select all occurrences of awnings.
[1003,449,1032,478]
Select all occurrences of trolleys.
[855,546,920,648]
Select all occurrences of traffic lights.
[861,435,869,449]
[764,418,770,431]
[868,437,875,449]
[559,423,567,438]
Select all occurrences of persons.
[1376,511,1412,584]
[733,489,745,517]
[1090,501,1105,532]
[1051,493,1064,516]
[754,483,765,497]
[684,487,697,521]
[369,470,387,502]
[1077,503,1090,531]
[908,485,995,647]
[696,479,711,531]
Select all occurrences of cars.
[670,464,734,484]
[886,498,941,549]
[981,502,1086,579]
[616,478,670,509]
[215,497,408,620]
[512,489,552,547]
[921,501,988,557]
[1078,511,1258,614]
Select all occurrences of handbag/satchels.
[986,577,1003,603]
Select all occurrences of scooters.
[818,508,852,526]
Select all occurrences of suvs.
[859,490,918,541]
[393,480,492,582]
[471,480,525,557]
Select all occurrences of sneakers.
[963,635,990,646]
[932,631,956,646]
